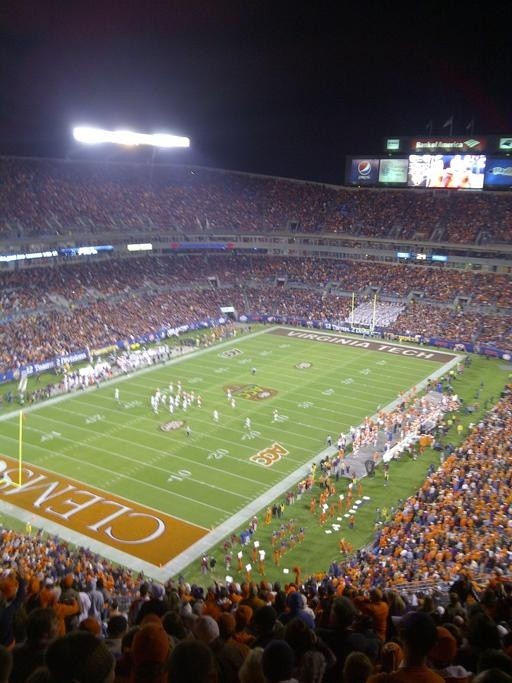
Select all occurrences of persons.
[1,154,512,683]
